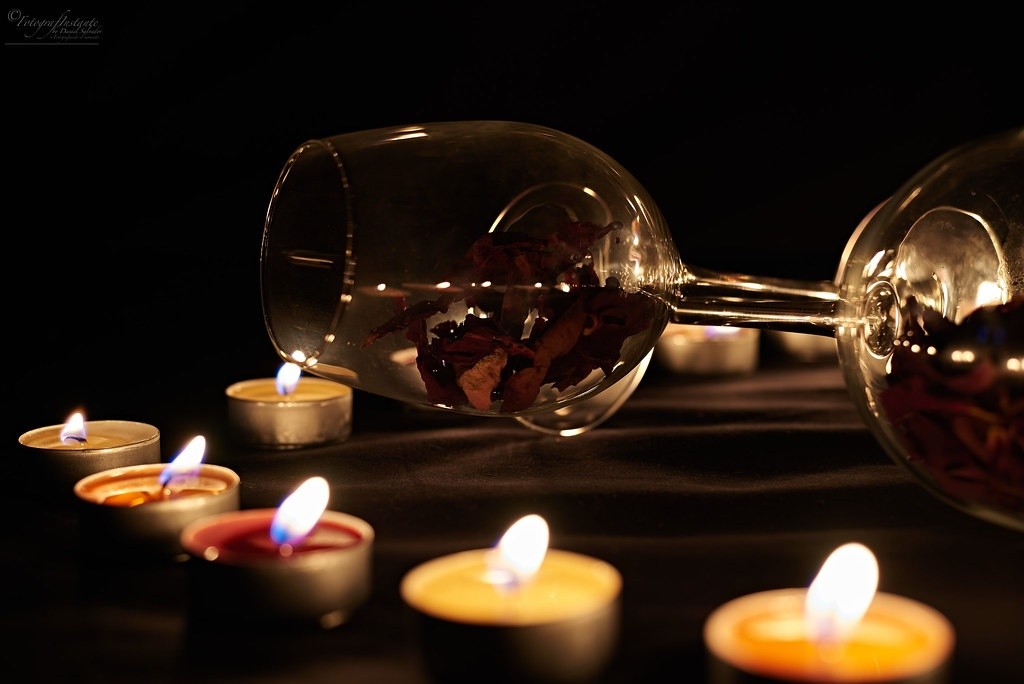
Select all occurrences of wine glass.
[259,120,1006,421]
[482,132,1024,538]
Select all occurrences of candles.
[700,587,958,683]
[179,506,375,632]
[227,377,351,449]
[72,464,241,556]
[18,419,163,493]
[401,548,624,684]
[657,328,759,375]
